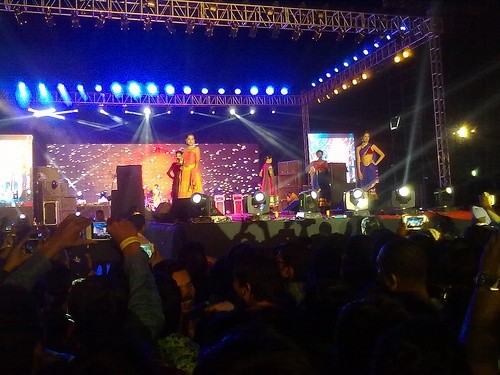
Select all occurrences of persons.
[260,155,279,219]
[0,191,500,375]
[167,150,183,199]
[356,131,385,198]
[306,149,333,218]
[152,184,161,208]
[178,133,204,198]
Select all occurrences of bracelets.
[120,236,140,250]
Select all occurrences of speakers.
[111,164,145,224]
[154,198,222,219]
[0,206,34,233]
[35,166,76,226]
[277,160,347,213]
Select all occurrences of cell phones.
[403,215,425,231]
[25,238,43,255]
[86,222,112,241]
[139,244,154,258]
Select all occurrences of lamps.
[248,24,257,38]
[190,186,456,217]
[401,29,414,39]
[142,16,153,32]
[120,14,130,31]
[354,29,367,45]
[43,10,57,28]
[70,10,81,28]
[379,30,387,40]
[317,48,413,105]
[228,23,239,39]
[203,21,215,38]
[335,28,346,44]
[291,27,303,43]
[13,7,31,28]
[184,18,195,35]
[311,27,323,44]
[26,104,277,118]
[94,14,105,31]
[165,18,176,35]
[270,24,280,40]
